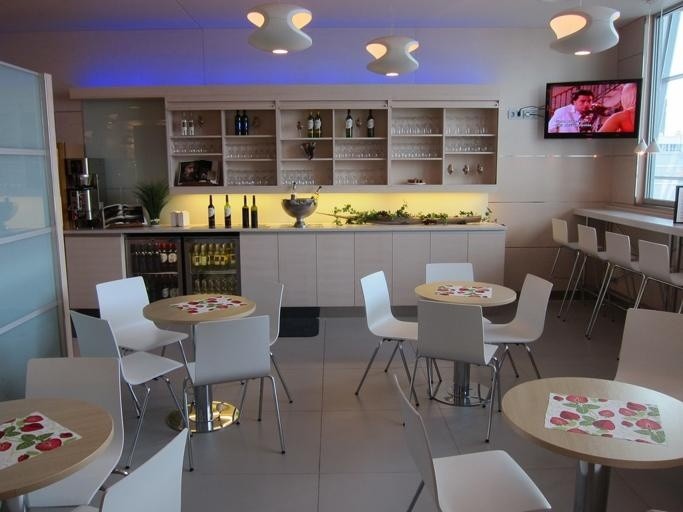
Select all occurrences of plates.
[405,181,428,185]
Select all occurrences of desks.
[500,377,681,511]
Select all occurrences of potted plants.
[129,179,174,225]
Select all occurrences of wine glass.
[391,114,494,158]
[333,141,385,184]
[172,141,219,153]
[280,198,316,228]
[447,163,486,185]
[225,143,275,186]
[280,168,314,185]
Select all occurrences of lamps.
[631,1,649,157]
[547,1,624,58]
[365,2,422,76]
[643,0,665,156]
[243,0,315,56]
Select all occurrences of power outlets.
[507,109,523,121]
[523,108,538,119]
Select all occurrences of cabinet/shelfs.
[238,224,505,307]
[273,100,390,195]
[164,99,276,193]
[64,227,125,311]
[122,225,240,305]
[385,98,497,193]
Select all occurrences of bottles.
[207,195,216,228]
[241,195,258,229]
[367,109,373,136]
[234,108,248,135]
[181,111,193,136]
[309,185,322,201]
[129,239,237,302]
[308,110,321,138]
[223,195,231,227]
[289,182,297,200]
[346,109,353,137]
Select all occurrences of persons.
[598,84,637,136]
[548,89,600,137]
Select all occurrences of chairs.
[542,205,680,361]
[613,308,681,400]
[354,262,553,442]
[393,373,551,511]
[1,276,292,508]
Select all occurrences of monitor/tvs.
[543,78,643,139]
